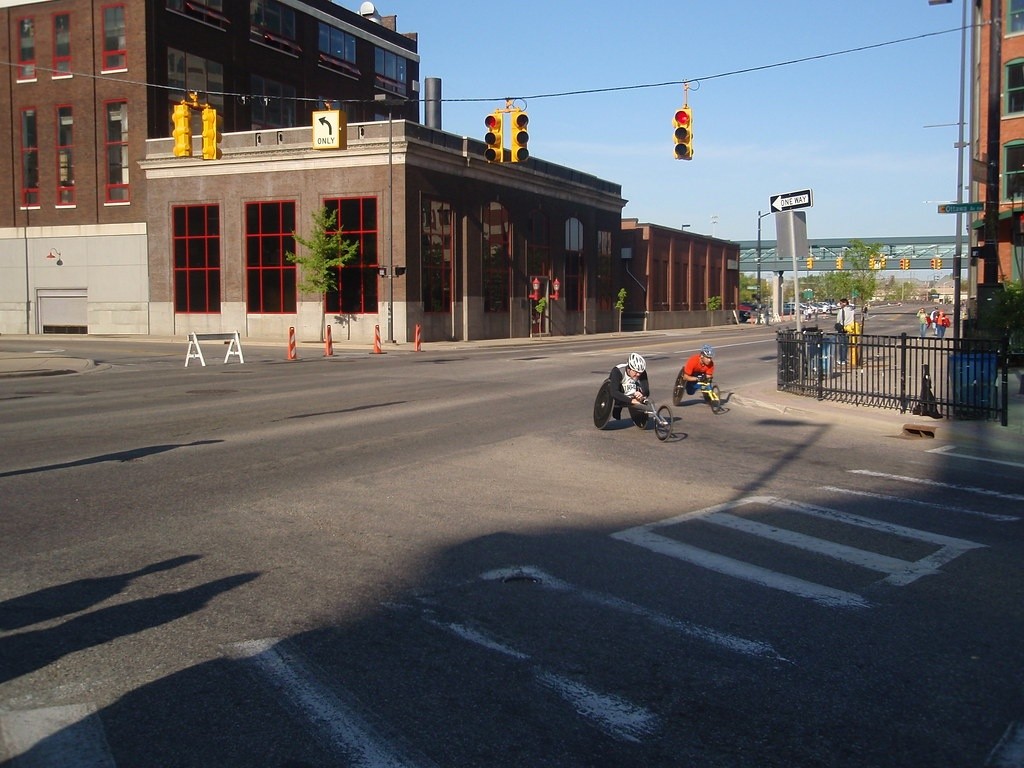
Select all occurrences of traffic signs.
[768,188,813,215]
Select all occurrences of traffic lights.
[484,113,504,163]
[202,109,224,160]
[905,259,910,270]
[673,109,694,161]
[930,259,935,269]
[881,258,887,269]
[869,258,875,269]
[899,259,904,270]
[937,259,943,270]
[836,258,843,270]
[806,258,813,269]
[511,112,529,163]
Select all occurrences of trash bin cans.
[947,350,997,418]
[802,330,823,378]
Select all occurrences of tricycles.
[673,366,721,415]
[593,378,674,443]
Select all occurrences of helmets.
[700,343,714,358]
[628,353,646,373]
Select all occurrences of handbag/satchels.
[834,322,845,333]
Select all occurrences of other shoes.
[837,359,846,365]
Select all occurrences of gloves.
[698,374,706,382]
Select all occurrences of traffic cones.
[410,324,426,352]
[284,327,303,361]
[369,325,387,354]
[323,325,335,357]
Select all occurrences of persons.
[754,307,758,324]
[760,307,766,324]
[930,306,940,335]
[864,301,870,320]
[803,307,808,321]
[836,299,854,365]
[608,352,650,421]
[935,310,949,338]
[808,305,813,320]
[683,344,715,381]
[768,305,773,316]
[916,307,929,337]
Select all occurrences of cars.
[887,301,902,307]
[737,299,868,322]
